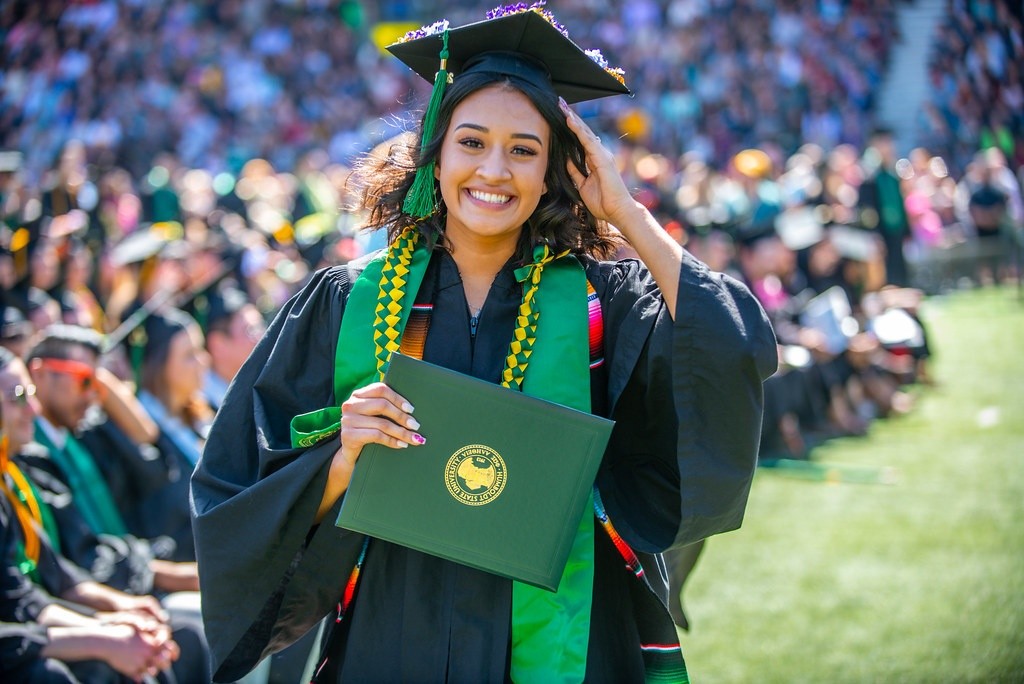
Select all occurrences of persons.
[365,0,1024,455]
[0,0,363,684]
[188,7,778,684]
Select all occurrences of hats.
[384,0,633,217]
[99,260,250,396]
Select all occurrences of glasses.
[5,381,38,406]
[28,356,107,398]
